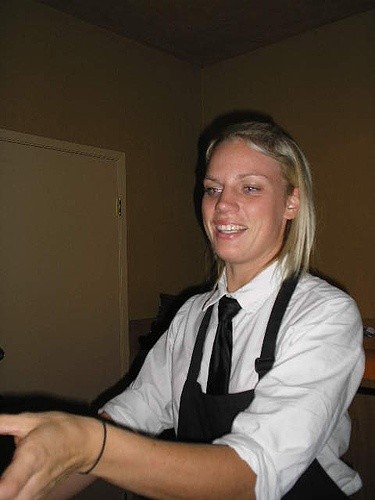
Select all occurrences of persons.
[0,122,366,500]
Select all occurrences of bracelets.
[78,420,107,475]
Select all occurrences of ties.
[206,294,242,396]
